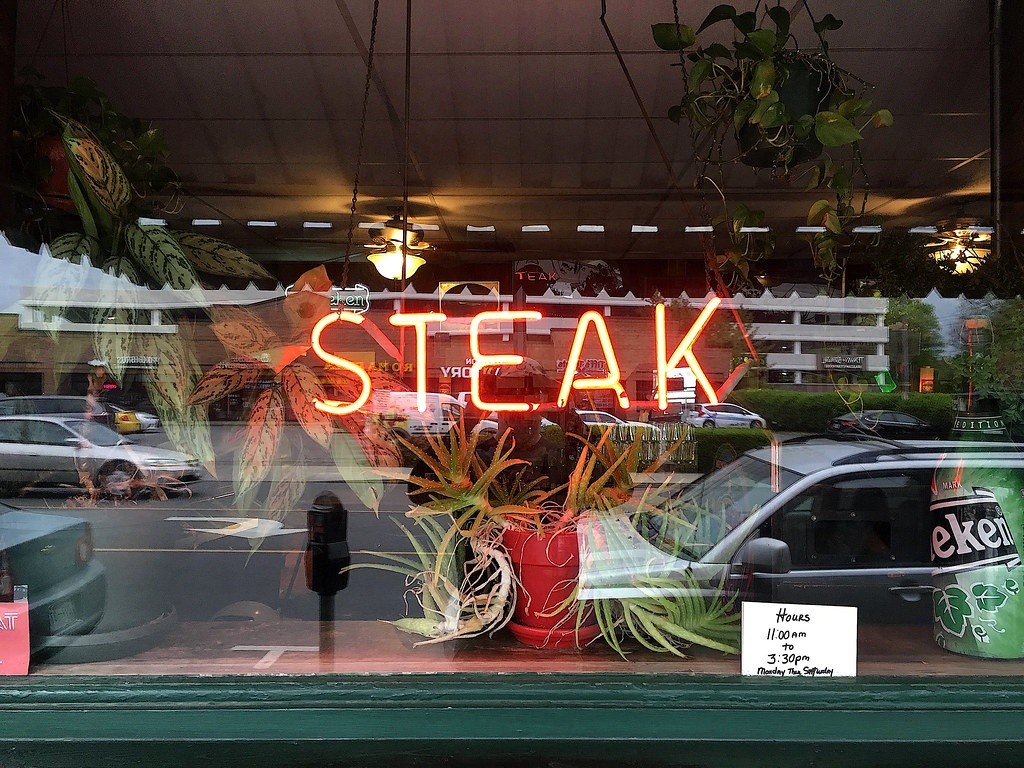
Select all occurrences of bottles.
[930,317,1024,658]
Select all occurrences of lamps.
[366,216,426,280]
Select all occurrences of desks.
[37,619,1023,676]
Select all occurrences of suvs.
[572,437,1024,629]
[681,403,767,430]
[0,395,117,432]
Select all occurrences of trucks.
[457,391,563,432]
[362,389,500,470]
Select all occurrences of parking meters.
[304,490,352,673]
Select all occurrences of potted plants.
[386,400,684,629]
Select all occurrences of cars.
[539,410,663,439]
[825,410,944,441]
[107,403,160,435]
[0,414,204,501]
[0,502,107,669]
